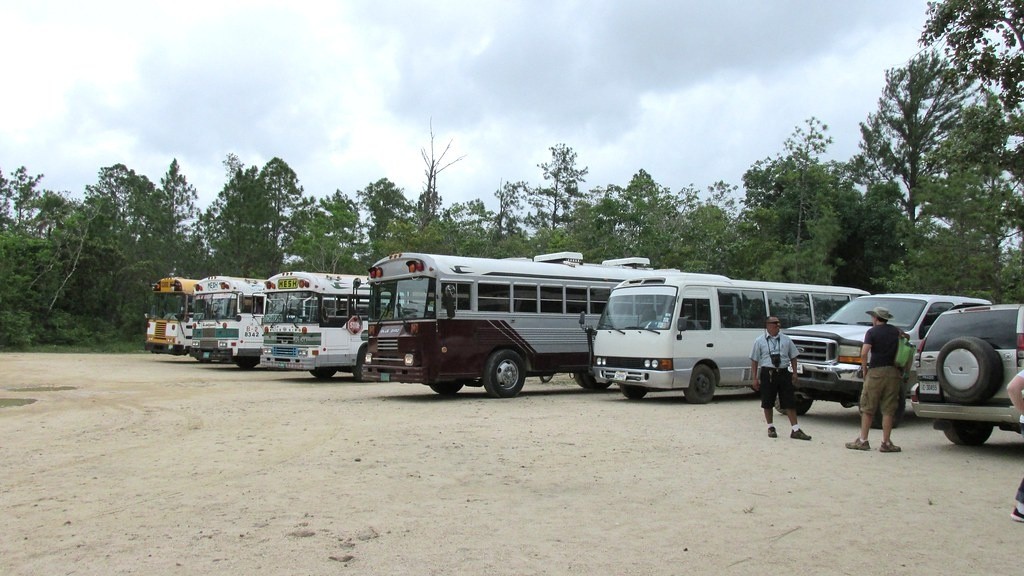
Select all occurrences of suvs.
[777,294,993,429]
[909,304,1023,446]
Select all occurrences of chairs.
[776,313,792,328]
[750,314,764,327]
[726,314,742,328]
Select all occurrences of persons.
[1005,369,1024,522]
[749,315,812,441]
[846,307,904,450]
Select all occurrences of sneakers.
[880,439,901,452]
[846,438,870,450]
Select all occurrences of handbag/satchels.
[894,329,917,368]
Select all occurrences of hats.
[866,307,895,321]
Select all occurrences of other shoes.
[767,427,778,438]
[1010,508,1024,522]
[791,429,812,441]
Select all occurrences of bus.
[350,251,731,399]
[189,275,274,371]
[250,271,427,380]
[577,271,870,404]
[143,276,198,356]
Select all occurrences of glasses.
[767,321,780,325]
